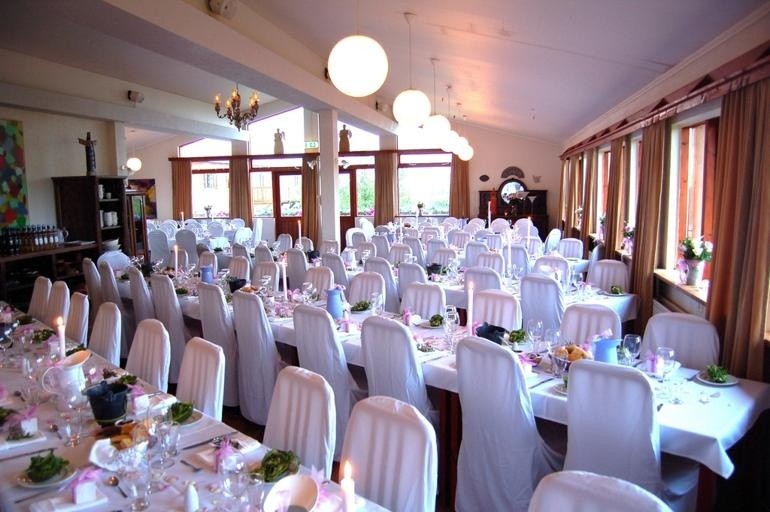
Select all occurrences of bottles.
[0,224,59,257]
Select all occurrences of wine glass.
[20,245,675,511]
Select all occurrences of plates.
[694,371,739,386]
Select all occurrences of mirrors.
[499,178,528,206]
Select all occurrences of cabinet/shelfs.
[478,190,550,241]
[52,176,131,263]
[127,189,148,262]
[0,243,99,312]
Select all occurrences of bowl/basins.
[105,211,119,227]
[102,237,123,252]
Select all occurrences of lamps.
[423,57,475,162]
[326,0,389,99]
[391,12,432,128]
[214,81,258,132]
[127,130,142,171]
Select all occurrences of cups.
[100,210,104,228]
[98,185,112,199]
